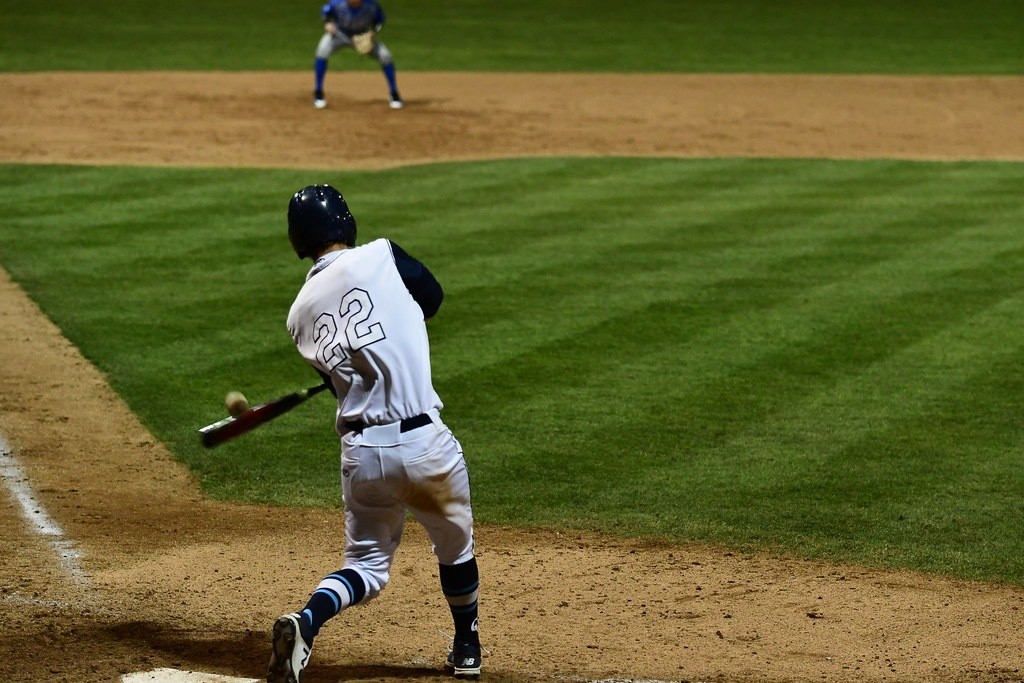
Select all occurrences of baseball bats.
[195,375,326,449]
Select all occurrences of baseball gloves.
[353,30,376,55]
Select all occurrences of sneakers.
[267,611,312,683]
[445,644,483,677]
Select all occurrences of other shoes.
[389,97,403,109]
[313,99,327,109]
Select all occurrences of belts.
[346,414,432,435]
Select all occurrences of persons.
[312,1,403,110]
[266,183,483,683]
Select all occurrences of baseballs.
[226,391,250,414]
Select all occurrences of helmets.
[287,185,357,259]
